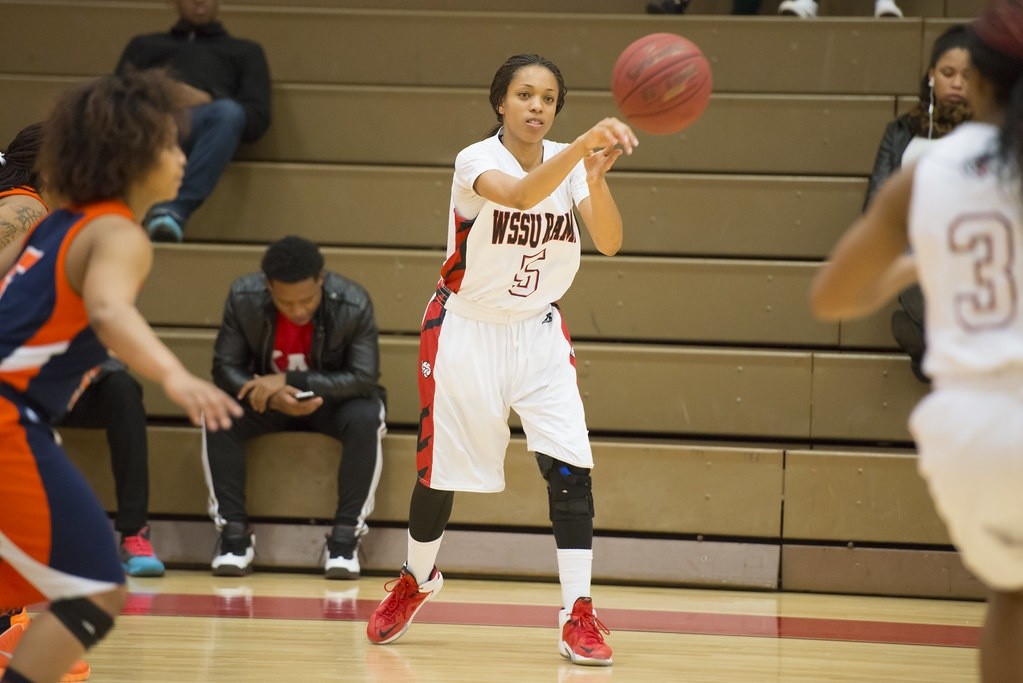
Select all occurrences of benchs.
[0,0,1023,600]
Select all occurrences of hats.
[971,0,1023,59]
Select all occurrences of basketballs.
[611,32,713,135]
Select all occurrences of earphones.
[929,73,935,87]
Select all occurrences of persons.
[200,234,388,578]
[862,22,979,383]
[367,54,639,667]
[0,74,246,683]
[645,0,904,18]
[112,0,272,242]
[807,0,1023,683]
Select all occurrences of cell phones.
[296,391,315,402]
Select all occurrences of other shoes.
[874,0,903,17]
[731,0,761,15]
[147,214,184,243]
[647,0,690,14]
[777,0,818,17]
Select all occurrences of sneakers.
[559,596,613,666]
[211,520,256,576]
[119,526,165,576]
[0,606,90,681]
[318,525,361,579]
[367,560,443,644]
